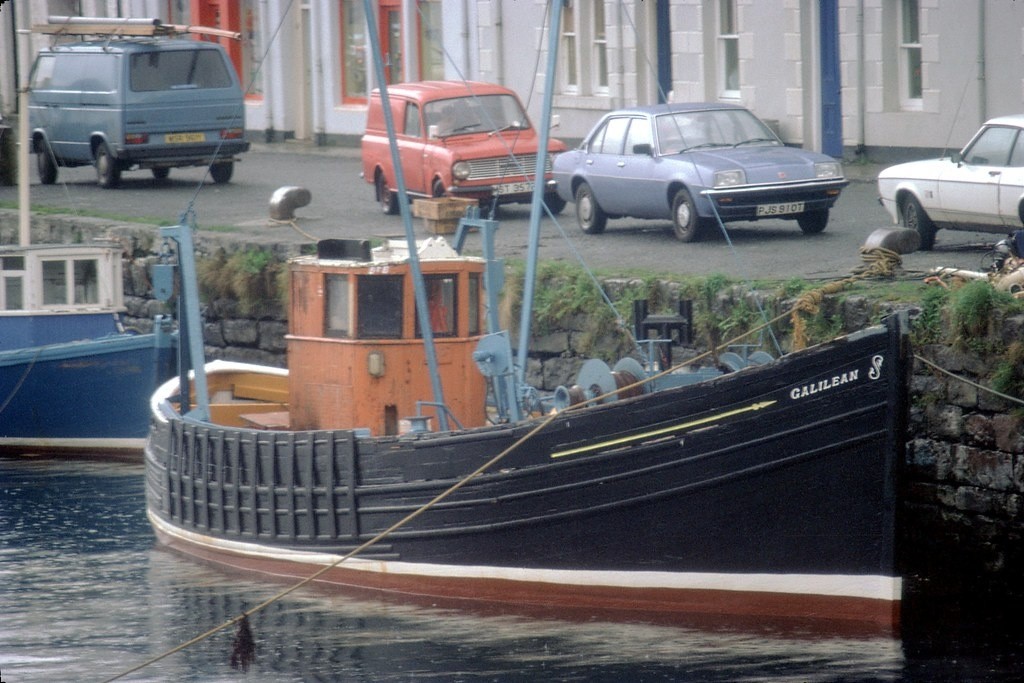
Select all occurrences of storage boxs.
[415,197,479,234]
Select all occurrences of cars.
[360,78,572,217]
[552,101,851,242]
[877,112,1024,250]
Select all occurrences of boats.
[141,1,918,659]
[0,226,192,465]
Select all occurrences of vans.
[22,36,251,190]
